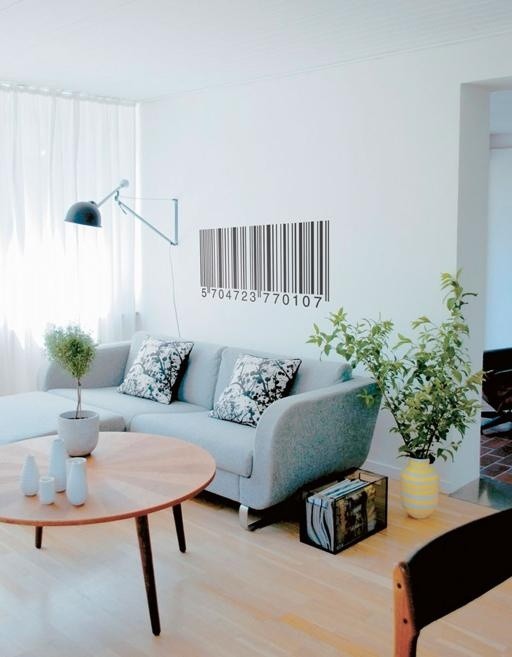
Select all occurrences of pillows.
[113,335,194,406]
[208,353,303,428]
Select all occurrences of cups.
[38,477,56,505]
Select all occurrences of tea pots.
[65,457,88,506]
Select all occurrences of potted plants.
[43,325,100,457]
[305,270,494,520]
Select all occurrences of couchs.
[43,331,384,531]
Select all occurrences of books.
[307,479,377,553]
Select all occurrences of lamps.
[63,179,178,247]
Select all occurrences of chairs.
[393,508,512,657]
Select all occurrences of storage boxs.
[297,467,388,555]
